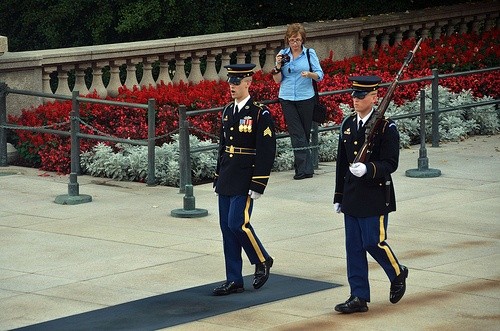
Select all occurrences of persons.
[272,23,324,179]
[212,63,276,294]
[333,76,408,313]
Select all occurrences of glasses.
[288,38,303,44]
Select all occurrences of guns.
[351,37,423,164]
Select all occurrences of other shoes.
[294,172,313,180]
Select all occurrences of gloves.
[334,203,342,214]
[248,190,261,200]
[349,162,367,178]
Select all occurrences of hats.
[348,75,382,98]
[223,63,256,84]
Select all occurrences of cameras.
[282,53,290,62]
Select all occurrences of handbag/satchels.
[312,101,327,123]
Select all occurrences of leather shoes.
[335,295,368,313]
[210,281,245,296]
[252,256,273,289]
[389,265,409,304]
